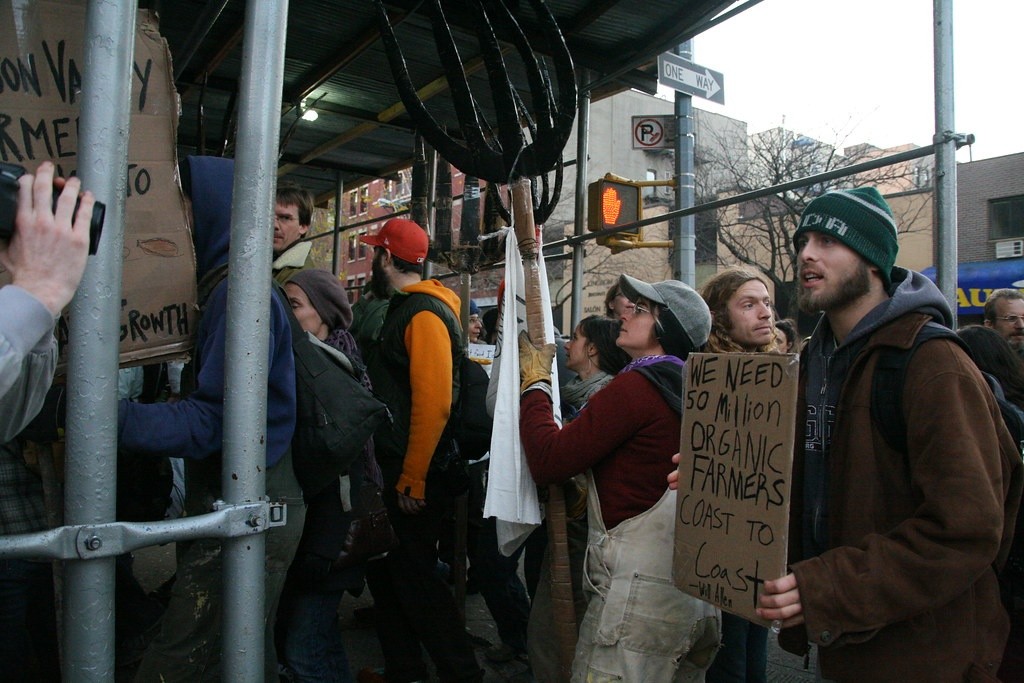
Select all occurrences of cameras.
[0,160,106,256]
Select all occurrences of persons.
[0,152,1024,683]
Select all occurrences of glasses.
[995,315,1024,322]
[634,302,664,339]
[275,215,300,224]
[615,292,624,298]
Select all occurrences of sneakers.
[485,642,519,662]
[358,666,424,683]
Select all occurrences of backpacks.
[799,323,1024,582]
[455,356,493,462]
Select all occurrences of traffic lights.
[588,172,642,254]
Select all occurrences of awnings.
[918,258,1024,319]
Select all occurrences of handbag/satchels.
[294,337,395,512]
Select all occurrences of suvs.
[473,297,560,348]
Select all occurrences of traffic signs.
[658,51,723,106]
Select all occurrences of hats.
[470,299,481,316]
[620,273,712,348]
[359,218,429,265]
[287,270,353,332]
[794,188,898,282]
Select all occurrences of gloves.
[518,330,555,393]
